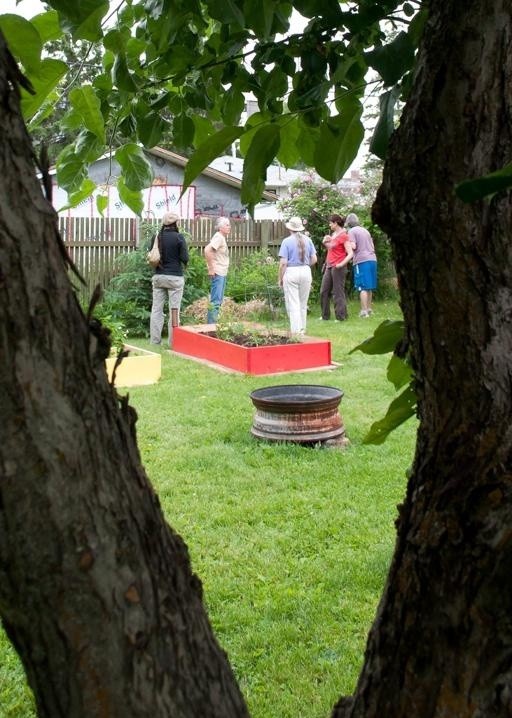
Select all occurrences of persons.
[150,212,188,347]
[204,217,230,324]
[278,217,317,336]
[319,214,377,321]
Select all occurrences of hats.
[285,217,305,231]
[163,212,178,226]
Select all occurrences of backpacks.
[146,234,161,268]
